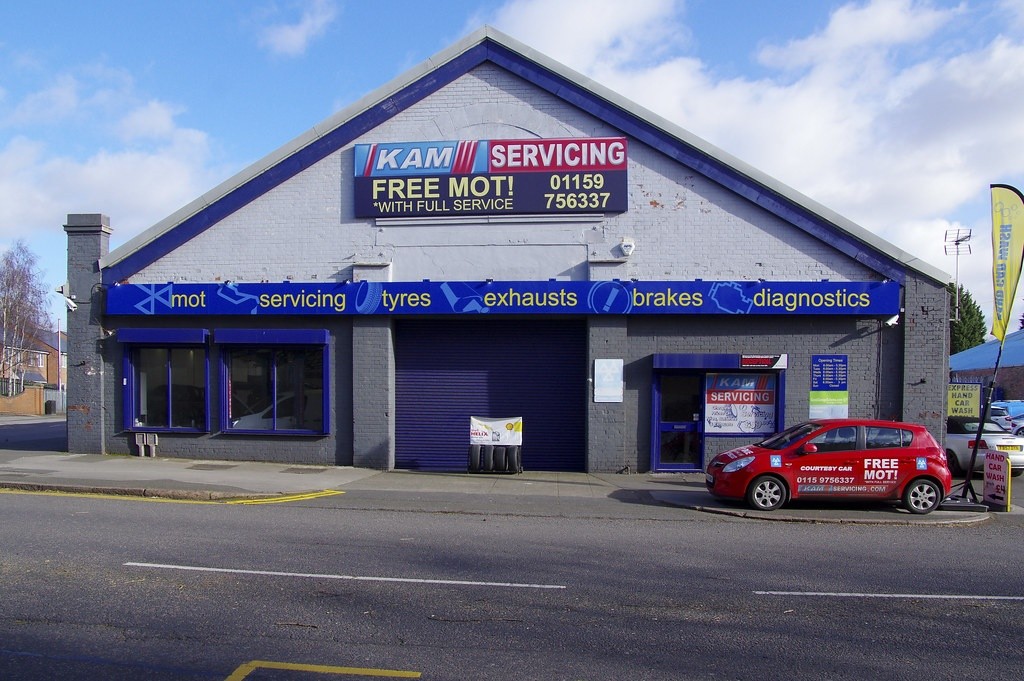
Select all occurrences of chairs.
[835,427,856,449]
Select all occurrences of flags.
[990,184,1024,342]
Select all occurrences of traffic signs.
[983,449,1009,506]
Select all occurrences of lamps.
[485,278,494,283]
[758,277,765,284]
[222,279,232,287]
[113,279,118,286]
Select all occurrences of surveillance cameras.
[884,313,900,327]
[64,297,77,310]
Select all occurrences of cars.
[232,387,324,430]
[1011,412,1024,436]
[147,384,206,427]
[990,406,1012,430]
[705,418,952,517]
[946,416,1024,476]
